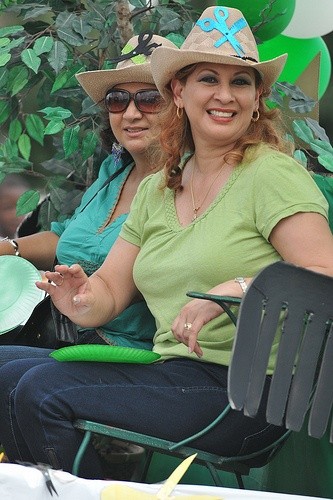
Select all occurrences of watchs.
[234,277,250,294]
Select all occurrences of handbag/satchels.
[15,294,79,349]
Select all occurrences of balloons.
[214,0,333,108]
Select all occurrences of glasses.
[105,87,174,114]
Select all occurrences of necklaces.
[0,5,333,480]
[189,152,242,220]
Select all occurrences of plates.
[0,255,43,334]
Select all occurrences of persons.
[0,34,179,465]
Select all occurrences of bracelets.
[6,239,20,257]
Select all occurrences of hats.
[75,29,181,115]
[150,5,287,111]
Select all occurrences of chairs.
[72,260,333,490]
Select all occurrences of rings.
[55,277,67,288]
[184,322,191,329]
